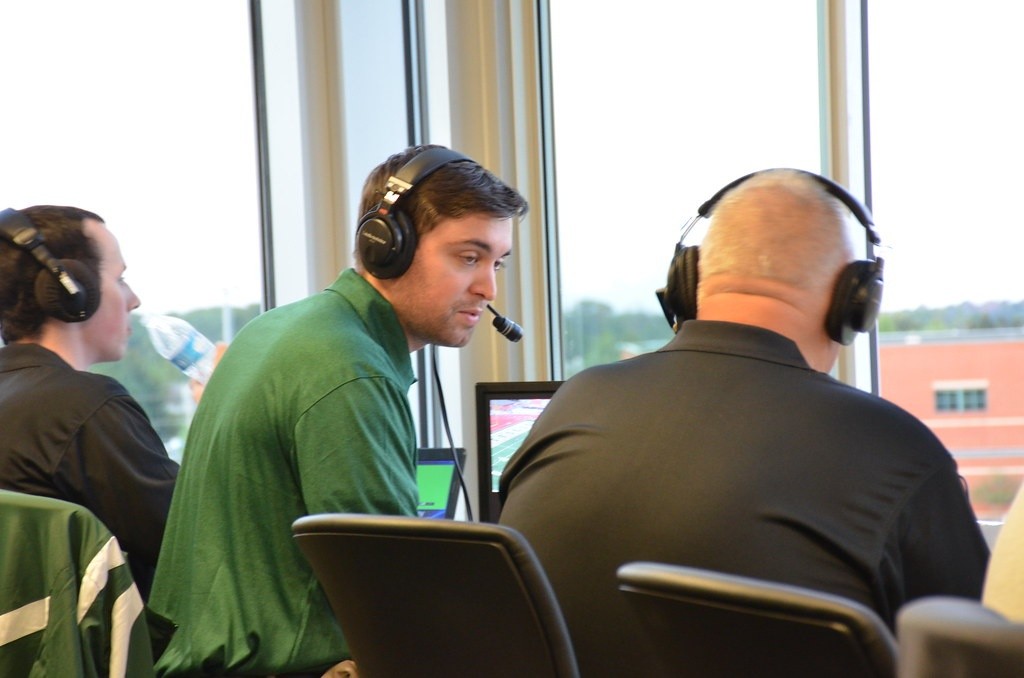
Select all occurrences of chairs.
[291,512,580,678]
[0,488,155,678]
[616,561,1024,678]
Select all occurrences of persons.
[147,145,530,678]
[0,204,230,663]
[495,170,991,642]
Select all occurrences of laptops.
[414,447,468,523]
[476,381,563,523]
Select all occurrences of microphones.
[485,305,524,343]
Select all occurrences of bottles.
[140,312,216,387]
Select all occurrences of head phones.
[654,169,885,347]
[0,208,102,323]
[355,148,478,279]
